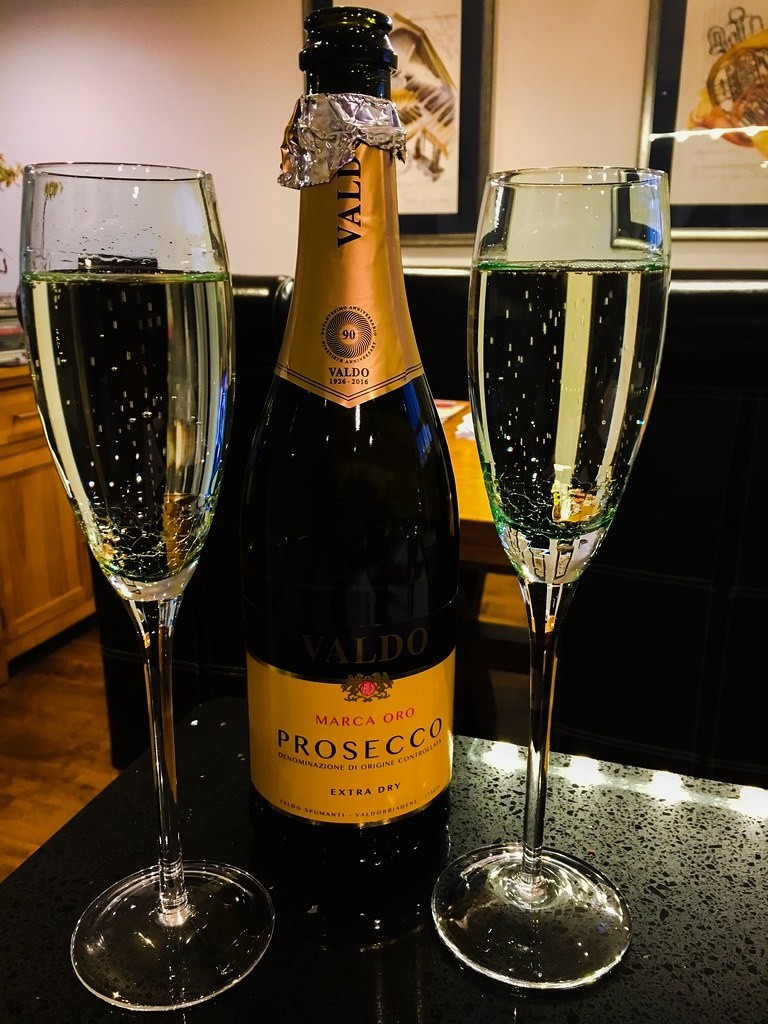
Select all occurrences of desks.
[1,732,768,1024]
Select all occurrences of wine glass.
[431,169,671,989]
[22,164,275,1012]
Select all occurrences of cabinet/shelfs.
[0,363,96,684]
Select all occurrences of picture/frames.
[633,1,768,241]
[303,0,496,248]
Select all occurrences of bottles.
[249,8,452,955]
